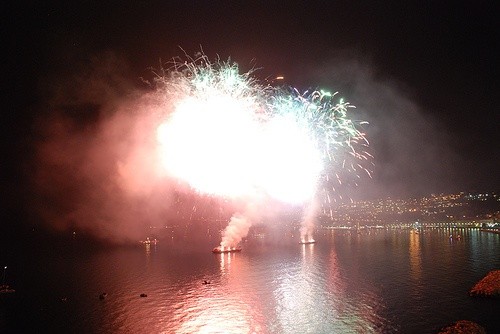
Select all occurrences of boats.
[136,237,160,245]
[298,238,316,244]
[213,245,242,254]
